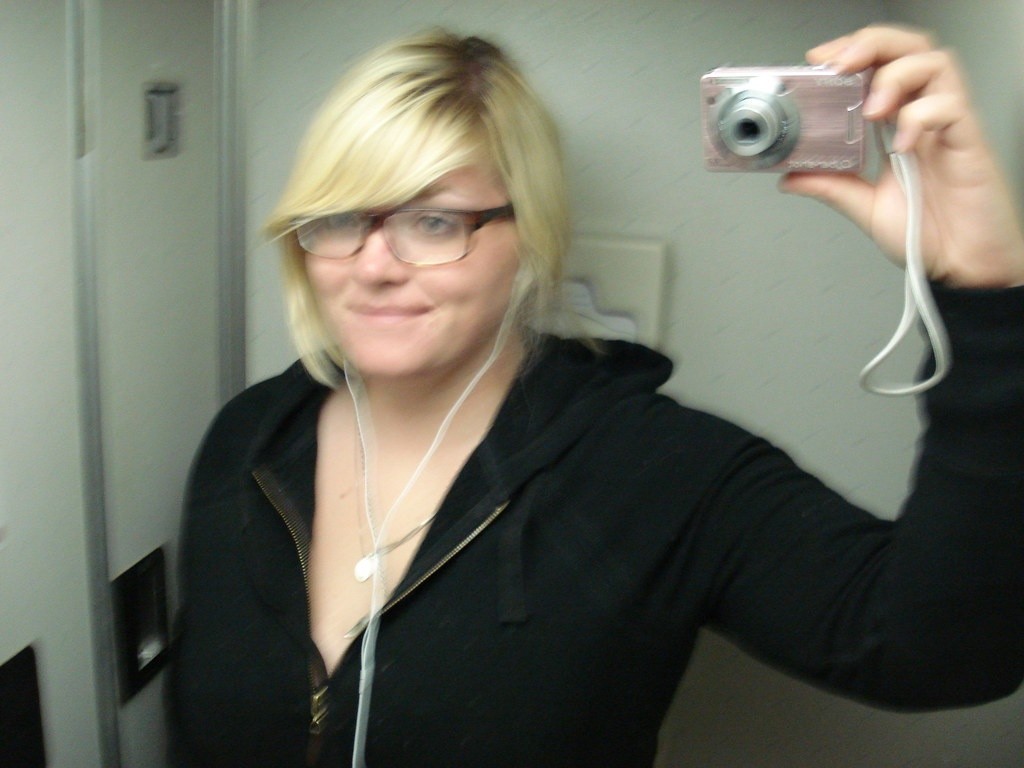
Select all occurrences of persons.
[165,25,1024,768]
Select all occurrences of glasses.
[293,204,514,267]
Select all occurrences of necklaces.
[340,394,440,585]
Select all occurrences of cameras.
[700,65,875,173]
